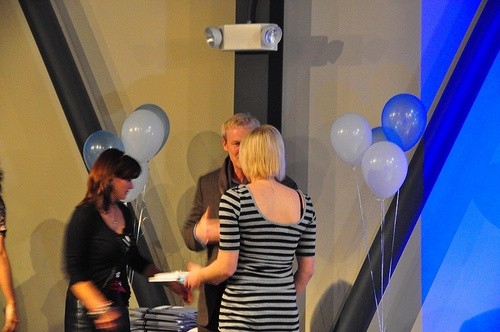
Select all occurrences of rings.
[13,319,21,324]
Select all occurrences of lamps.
[204,24,283,51]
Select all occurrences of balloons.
[83,109,165,204]
[329,93,428,200]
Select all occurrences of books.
[149,271,190,283]
[128,304,197,332]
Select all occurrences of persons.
[0,169,21,332]
[180,112,318,332]
[58,147,194,332]
